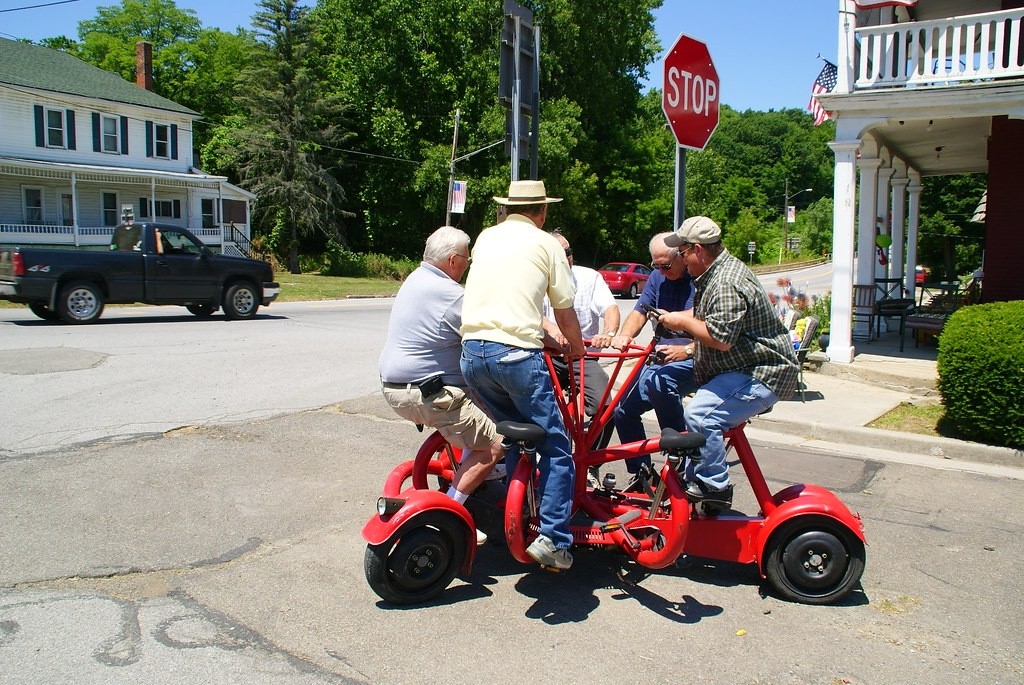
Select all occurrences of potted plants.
[819,328,830,352]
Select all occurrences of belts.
[382,381,420,389]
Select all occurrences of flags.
[807,59,837,126]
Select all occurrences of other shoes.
[626,466,661,492]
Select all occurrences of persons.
[543,232,621,487]
[379,226,506,545]
[459,180,587,568]
[647,216,801,512]
[611,232,700,485]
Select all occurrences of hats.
[492,180,563,205]
[665,216,721,247]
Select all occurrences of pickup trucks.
[0,205,279,324]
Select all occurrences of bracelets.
[604,331,614,337]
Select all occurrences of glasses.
[564,247,573,258]
[651,255,678,270]
[678,244,705,260]
[448,253,472,265]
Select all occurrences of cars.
[596,263,652,299]
[915,267,929,283]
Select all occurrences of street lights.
[446,132,533,227]
[784,188,812,257]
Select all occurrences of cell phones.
[640,302,663,319]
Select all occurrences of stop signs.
[661,32,720,151]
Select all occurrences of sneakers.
[683,484,735,508]
[522,488,540,519]
[474,528,488,546]
[526,534,573,570]
[587,467,601,491]
[484,463,507,481]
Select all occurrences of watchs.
[685,345,693,358]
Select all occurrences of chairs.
[852,278,978,352]
[785,308,821,404]
